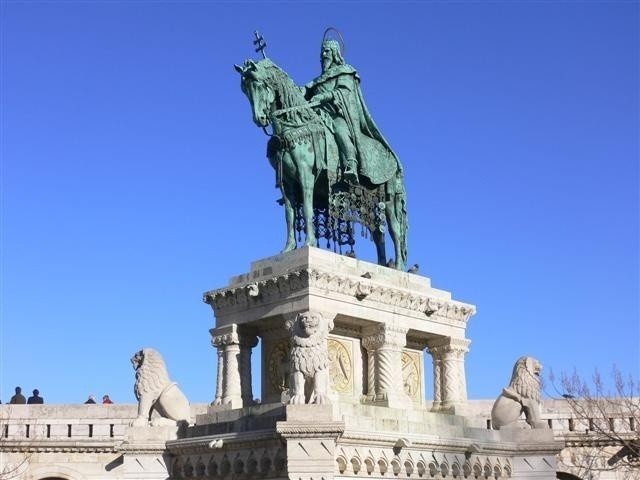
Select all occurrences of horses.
[234,58,409,272]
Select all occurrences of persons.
[26,389,43,404]
[102,395,113,404]
[294,39,403,186]
[10,387,26,404]
[84,395,96,404]
